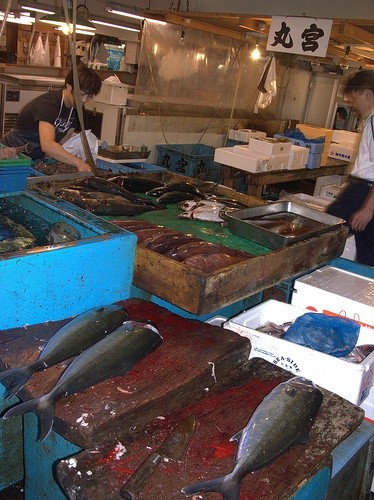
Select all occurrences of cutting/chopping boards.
[0,296,250,449]
[54,360,366,500]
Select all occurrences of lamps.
[0,0,165,37]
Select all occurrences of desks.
[220,157,349,198]
[0,74,66,135]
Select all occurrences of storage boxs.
[0,188,137,330]
[155,121,361,212]
[131,233,374,421]
[96,158,166,174]
[0,144,32,193]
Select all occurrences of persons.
[324,70,374,266]
[2,65,102,172]
[335,107,352,131]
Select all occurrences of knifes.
[121,414,196,500]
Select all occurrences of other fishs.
[2,317,170,445]
[101,217,260,278]
[180,196,243,233]
[179,373,324,500]
[48,165,181,217]
[0,301,129,406]
